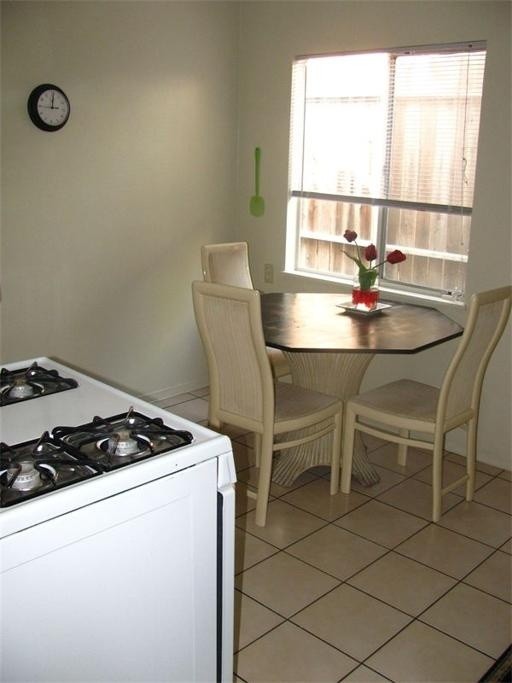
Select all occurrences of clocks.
[27,80,73,132]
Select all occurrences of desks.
[260,291,464,489]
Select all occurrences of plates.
[334,299,391,316]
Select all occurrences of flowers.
[339,227,407,290]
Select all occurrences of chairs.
[198,239,293,378]
[339,284,512,524]
[189,277,345,528]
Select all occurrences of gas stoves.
[0,355,238,539]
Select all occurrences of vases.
[334,286,392,318]
